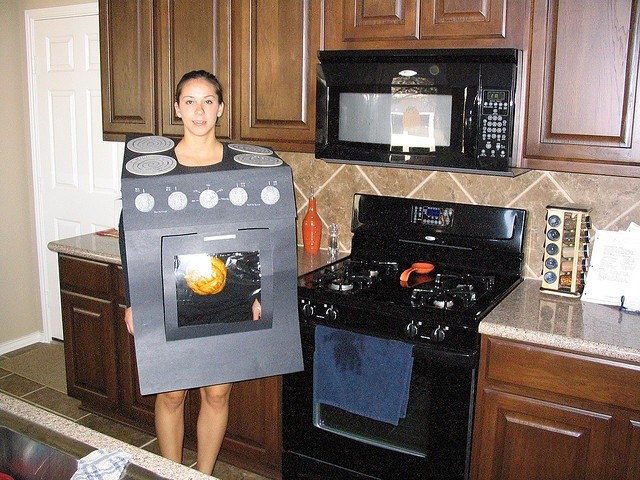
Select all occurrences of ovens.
[281,321,478,480]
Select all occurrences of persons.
[118,70,262,477]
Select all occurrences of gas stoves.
[296,193,527,354]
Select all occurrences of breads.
[184,255,228,297]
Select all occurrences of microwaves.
[314,47,531,178]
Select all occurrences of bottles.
[560,259,589,273]
[302,188,324,255]
[562,231,591,245]
[561,246,589,259]
[564,216,593,231]
[560,273,587,286]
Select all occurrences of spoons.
[401,275,433,289]
[400,262,435,283]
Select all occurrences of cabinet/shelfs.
[520,0,639,179]
[98,0,238,146]
[323,0,531,53]
[121,269,284,478]
[238,1,325,155]
[470,337,640,479]
[47,243,119,422]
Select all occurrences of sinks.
[0,427,135,480]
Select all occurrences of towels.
[70,449,131,480]
[313,326,415,427]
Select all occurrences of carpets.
[3,342,67,396]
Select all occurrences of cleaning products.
[302,185,322,254]
